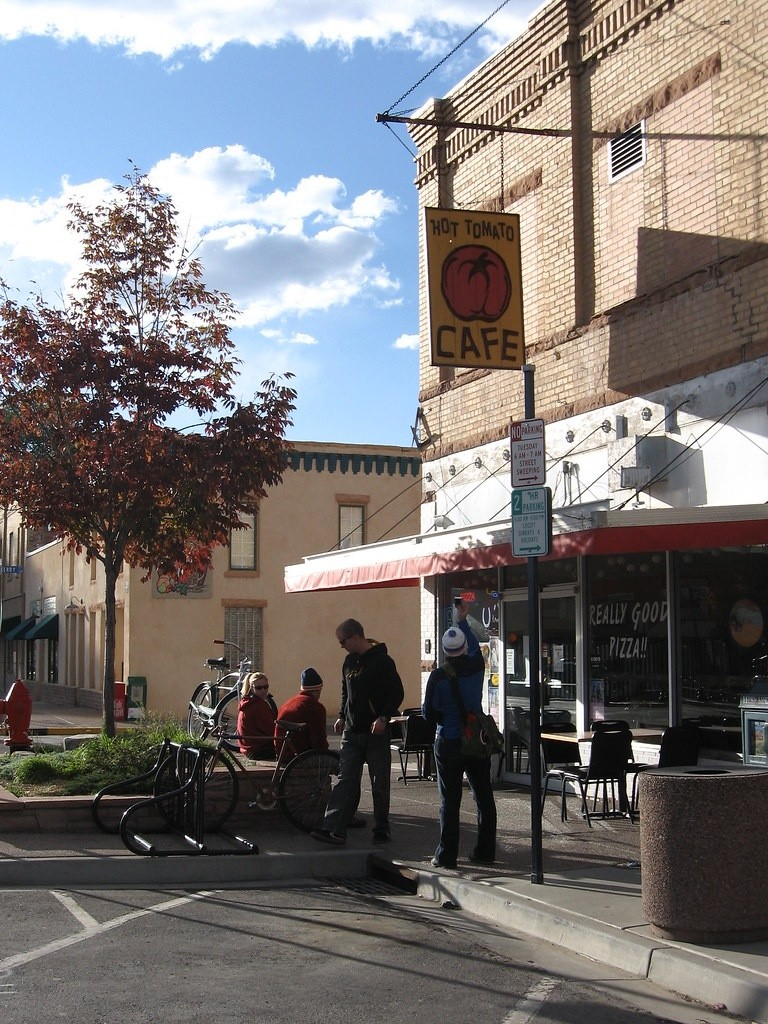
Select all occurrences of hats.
[442,627,467,656]
[301,668,324,691]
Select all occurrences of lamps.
[433,514,455,529]
[64,596,83,609]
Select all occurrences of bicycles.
[187,639,279,751]
[152,716,356,835]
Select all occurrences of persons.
[310,618,405,846]
[420,602,497,869]
[274,668,329,768]
[238,671,278,761]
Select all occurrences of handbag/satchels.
[460,714,503,757]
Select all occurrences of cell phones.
[454,597,463,609]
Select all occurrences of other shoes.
[347,817,366,828]
[468,854,492,865]
[431,857,446,868]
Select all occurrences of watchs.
[380,716,387,722]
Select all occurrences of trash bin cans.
[636,765,768,942]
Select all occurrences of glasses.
[253,685,269,690]
[339,637,352,645]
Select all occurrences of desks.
[389,715,435,781]
[540,727,664,820]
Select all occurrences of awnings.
[25,613,58,640]
[4,618,36,640]
[284,503,768,594]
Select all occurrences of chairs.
[539,723,592,827]
[630,726,699,811]
[561,727,635,827]
[590,720,648,809]
[509,707,532,773]
[390,707,434,786]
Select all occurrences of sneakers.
[372,830,391,844]
[311,829,345,844]
